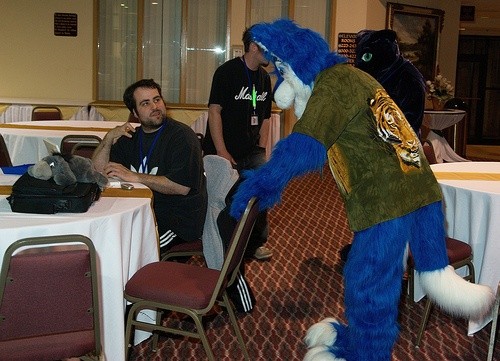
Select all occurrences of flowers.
[426,76,455,104]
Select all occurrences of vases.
[430,94,447,110]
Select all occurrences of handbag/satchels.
[6,170,102,215]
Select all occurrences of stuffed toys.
[27,152,109,189]
[231,18,494,361]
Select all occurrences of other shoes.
[252,245,274,259]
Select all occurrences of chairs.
[0,234,106,361]
[402,237,475,348]
[32,106,62,121]
[123,197,259,361]
[0,134,14,168]
[61,135,103,160]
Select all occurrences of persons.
[205,26,273,260]
[92,79,208,255]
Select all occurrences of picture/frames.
[384,2,446,82]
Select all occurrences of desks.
[0,175,165,360]
[0,120,142,185]
[428,161,500,336]
[423,110,468,135]
